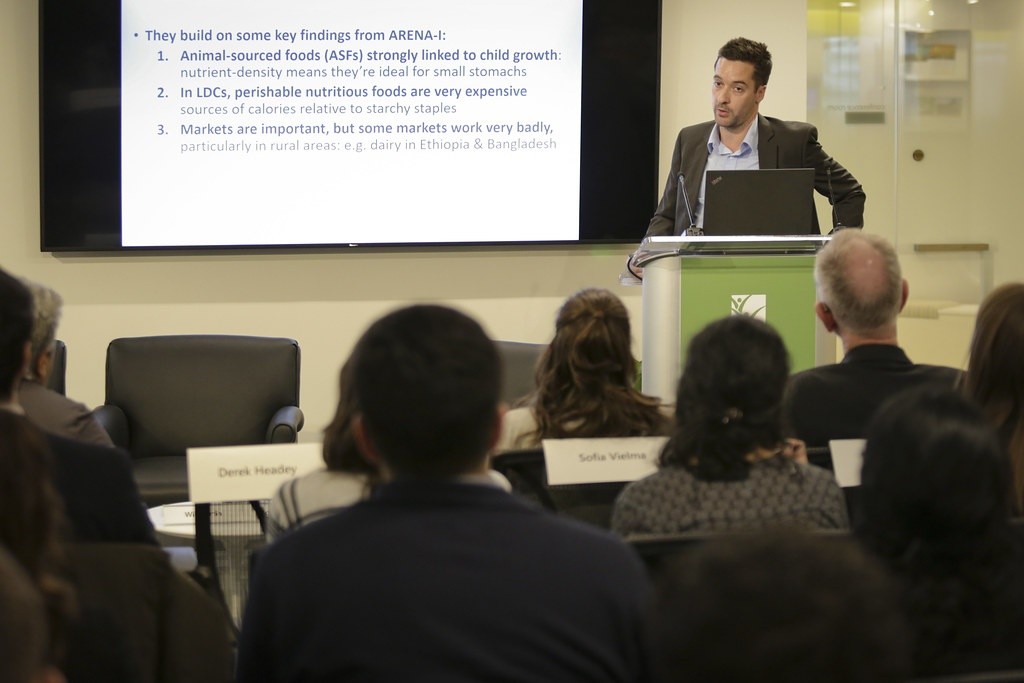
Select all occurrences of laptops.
[703,167,815,235]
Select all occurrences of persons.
[628,36,867,272]
[1,229,1024,683]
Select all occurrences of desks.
[146,502,268,594]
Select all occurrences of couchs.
[93,334,304,508]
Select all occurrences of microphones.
[677,172,704,236]
[823,159,847,234]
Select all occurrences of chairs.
[492,449,626,528]
